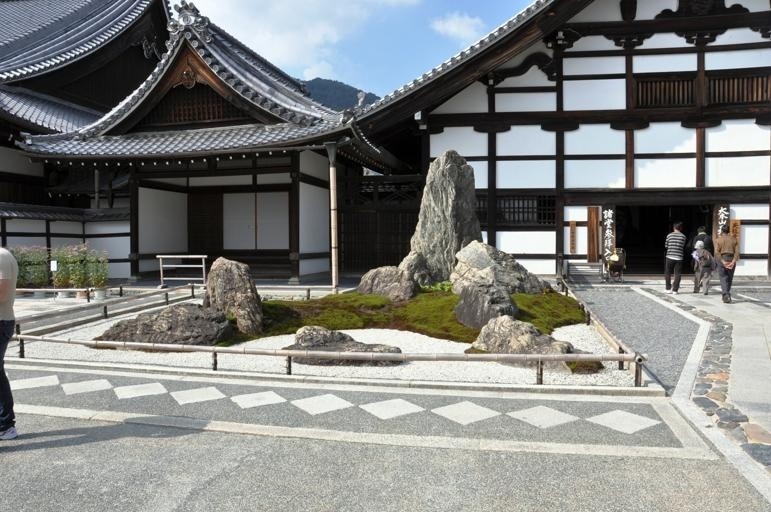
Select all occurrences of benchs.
[566,261,606,282]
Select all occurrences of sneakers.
[0,425,18,439]
[722,293,731,303]
[693,288,708,295]
[664,288,678,294]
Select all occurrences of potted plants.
[6,241,109,300]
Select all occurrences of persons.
[0,246,19,440]
[690,225,714,255]
[690,240,714,295]
[664,220,687,296]
[714,224,739,302]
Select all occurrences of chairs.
[607,248,626,284]
